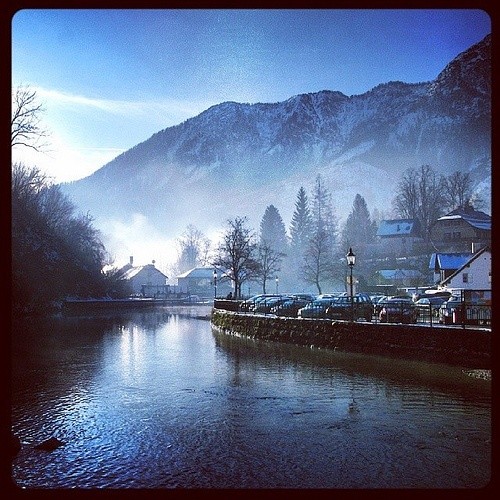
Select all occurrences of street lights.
[248,284,251,296]
[274,277,279,294]
[212,269,218,298]
[345,248,359,322]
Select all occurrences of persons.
[224,290,238,301]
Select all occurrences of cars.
[376,296,414,316]
[248,296,280,310]
[270,300,310,318]
[299,299,331,319]
[238,293,282,311]
[379,299,417,323]
[411,290,451,302]
[369,296,383,304]
[413,297,445,317]
[261,298,291,313]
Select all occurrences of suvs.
[325,296,374,322]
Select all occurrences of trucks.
[439,289,464,318]
